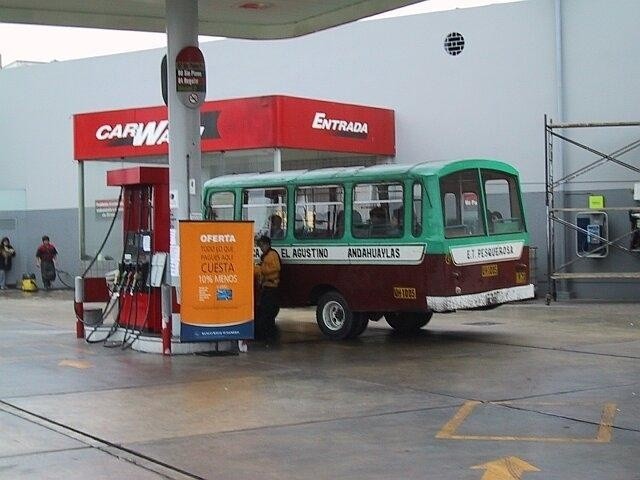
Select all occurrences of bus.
[201,158,535,341]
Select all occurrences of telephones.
[587,224,600,244]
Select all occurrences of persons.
[250,234,283,350]
[387,207,405,237]
[478,209,493,232]
[264,214,284,238]
[491,210,505,224]
[367,207,390,235]
[0,237,17,290]
[35,234,59,292]
